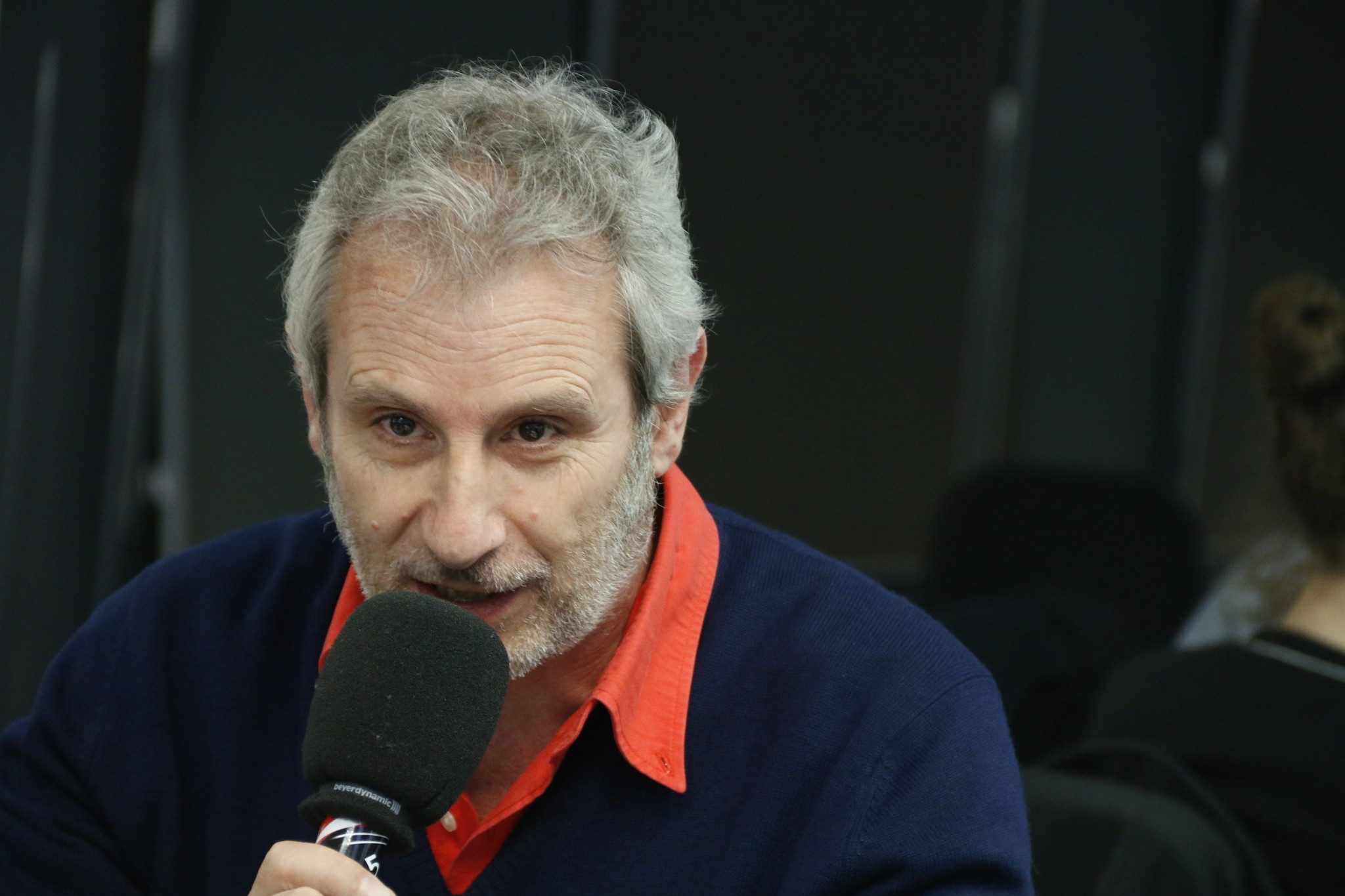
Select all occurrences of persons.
[1067,277,1345,896]
[0,56,1034,896]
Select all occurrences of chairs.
[911,462,1209,646]
[1022,764,1247,895]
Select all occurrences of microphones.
[296,589,511,878]
